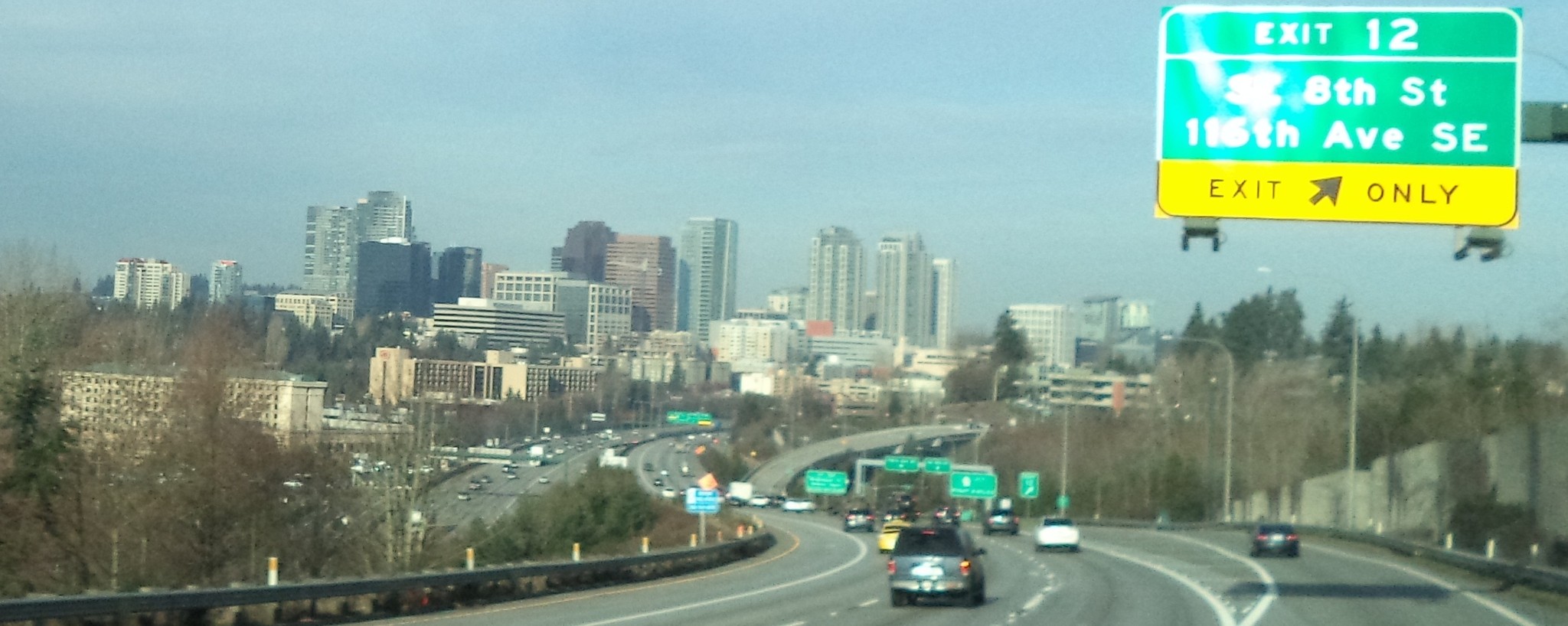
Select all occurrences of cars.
[723,493,819,514]
[886,527,987,609]
[844,495,961,552]
[592,428,624,451]
[547,438,593,457]
[282,480,303,488]
[370,459,436,475]
[500,460,521,479]
[1034,516,1082,553]
[455,474,490,501]
[538,474,550,486]
[1249,525,1300,558]
[984,510,1021,537]
[688,431,718,445]
[643,462,691,495]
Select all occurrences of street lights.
[1161,335,1235,522]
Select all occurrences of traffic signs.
[949,472,998,498]
[804,471,850,496]
[1156,9,1522,230]
[1018,472,1040,498]
[884,454,921,472]
[923,456,954,473]
